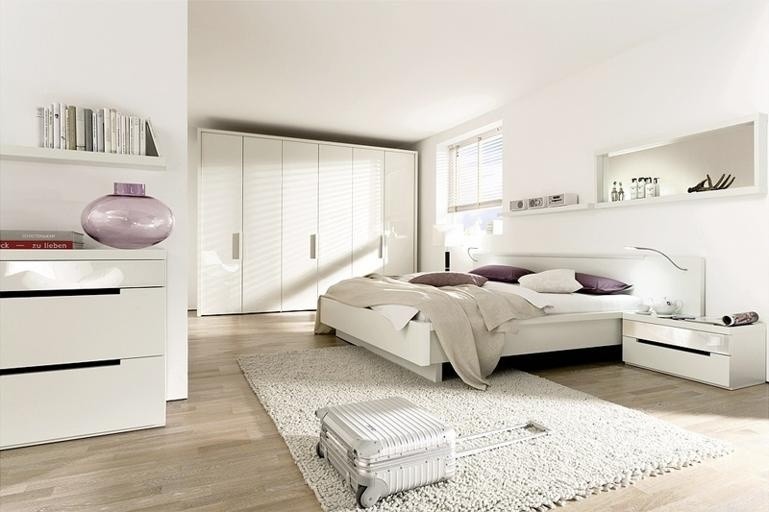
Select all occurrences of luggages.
[314,397,457,508]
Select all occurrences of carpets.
[235,344,738,511]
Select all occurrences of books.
[35,102,160,156]
[0,230,84,250]
[685,310,759,327]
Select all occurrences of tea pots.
[653,301,681,314]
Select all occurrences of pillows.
[576,272,632,296]
[470,263,534,283]
[516,269,582,295]
[408,272,487,290]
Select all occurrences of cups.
[637,304,650,312]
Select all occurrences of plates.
[657,314,676,318]
[636,311,651,315]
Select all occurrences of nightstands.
[621,312,764,391]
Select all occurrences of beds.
[317,272,681,385]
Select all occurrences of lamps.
[623,245,690,273]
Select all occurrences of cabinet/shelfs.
[195,128,419,318]
[0,248,168,454]
